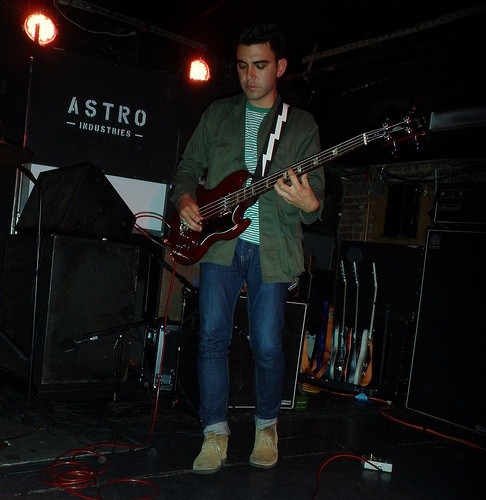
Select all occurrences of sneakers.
[191,431,228,475]
[247,423,278,470]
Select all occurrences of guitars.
[344,258,364,388]
[327,259,346,381]
[167,106,430,267]
[359,260,378,389]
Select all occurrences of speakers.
[15,162,137,243]
[401,227,486,448]
[171,287,307,412]
[33,231,163,402]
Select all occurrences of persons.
[170,24,325,475]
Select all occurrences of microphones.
[64,335,98,352]
